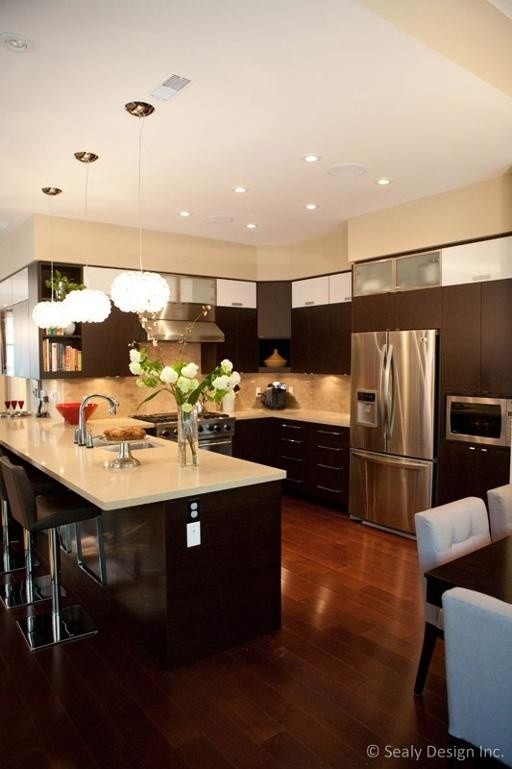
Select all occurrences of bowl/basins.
[57,404,98,425]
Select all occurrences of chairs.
[0,448,102,653]
[412,484,512,769]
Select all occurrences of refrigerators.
[348,329,436,539]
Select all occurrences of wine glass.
[5,400,25,417]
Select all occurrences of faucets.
[77,392,117,446]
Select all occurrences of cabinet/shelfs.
[216,270,351,376]
[269,417,349,508]
[39,260,140,380]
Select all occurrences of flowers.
[127,304,241,467]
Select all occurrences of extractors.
[139,301,226,344]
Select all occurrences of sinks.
[99,440,166,452]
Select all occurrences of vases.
[177,403,199,467]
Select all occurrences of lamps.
[32,100,171,331]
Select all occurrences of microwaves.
[444,394,512,447]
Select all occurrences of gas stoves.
[129,410,236,441]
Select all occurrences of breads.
[103,425,146,440]
